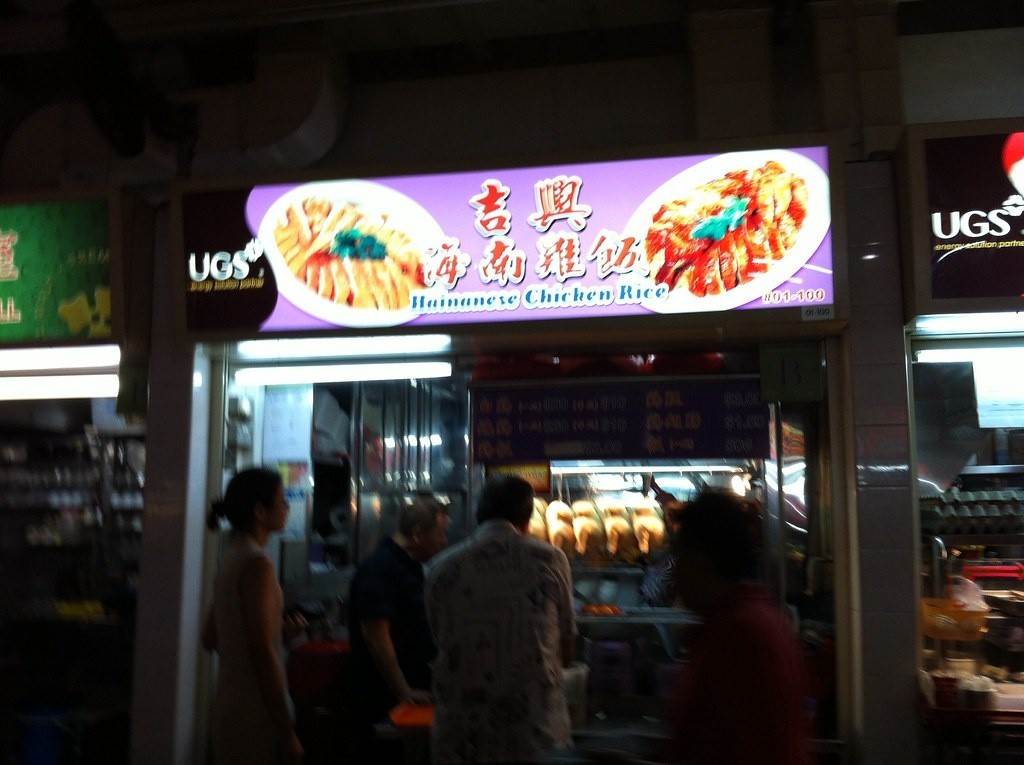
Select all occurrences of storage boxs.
[921,598,992,642]
[992,683,1024,709]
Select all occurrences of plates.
[621,148,831,315]
[258,179,447,329]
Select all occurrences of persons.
[426,474,579,765]
[344,497,449,765]
[200,468,309,765]
[661,494,818,765]
